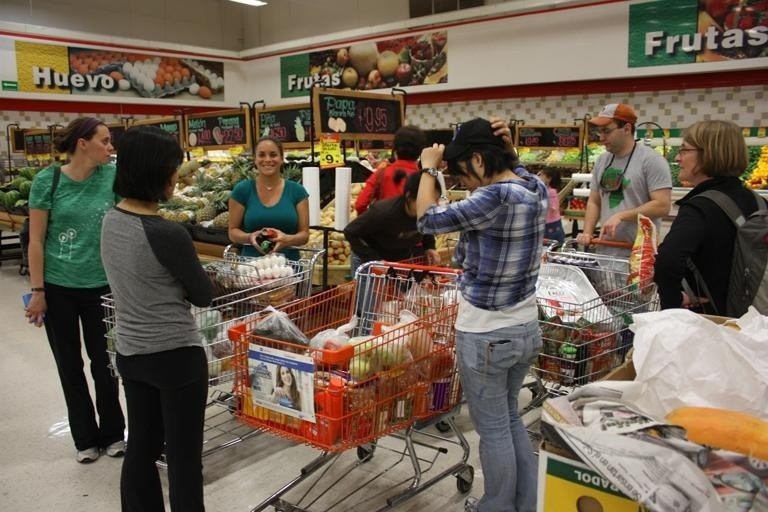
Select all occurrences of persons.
[21,117,127,464]
[270,366,302,411]
[654,120,768,317]
[343,124,450,336]
[535,167,565,251]
[100,124,213,512]
[574,103,673,386]
[416,115,551,511]
[226,135,310,295]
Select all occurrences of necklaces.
[257,176,282,191]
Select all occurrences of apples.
[336,48,348,66]
[394,63,411,82]
[342,67,359,87]
[369,70,379,86]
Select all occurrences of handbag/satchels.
[20,166,60,267]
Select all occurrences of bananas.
[648,406,768,462]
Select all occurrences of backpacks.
[687,189,768,317]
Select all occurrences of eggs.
[234,254,294,278]
[69,48,224,98]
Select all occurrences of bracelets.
[31,288,45,292]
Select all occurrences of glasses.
[679,147,697,153]
[600,168,623,192]
[596,127,617,136]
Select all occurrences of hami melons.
[377,51,399,78]
[347,43,377,74]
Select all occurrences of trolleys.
[524,238,660,458]
[98,243,327,471]
[228,261,475,512]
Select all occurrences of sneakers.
[465,497,482,512]
[76,441,127,463]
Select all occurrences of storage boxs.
[536,312,768,512]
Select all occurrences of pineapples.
[158,157,301,229]
[292,116,305,142]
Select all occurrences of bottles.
[559,347,577,387]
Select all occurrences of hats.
[589,103,637,125]
[442,117,505,160]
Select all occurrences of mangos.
[307,181,366,268]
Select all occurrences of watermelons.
[0,166,45,209]
